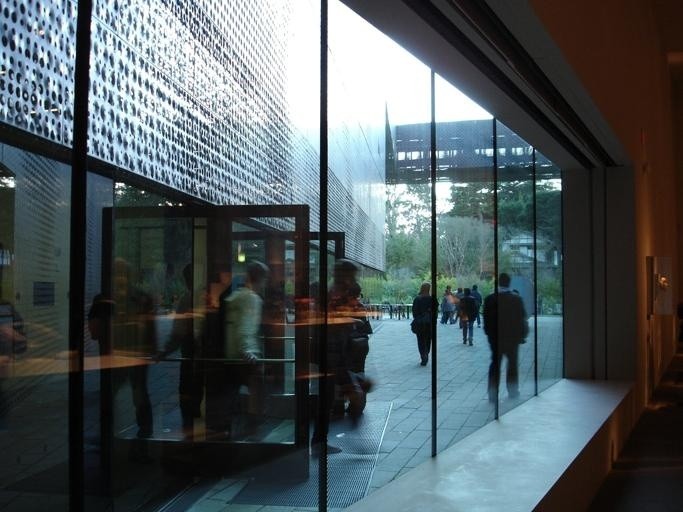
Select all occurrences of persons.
[88,257,367,456]
[0,300,27,359]
[412,273,528,403]
